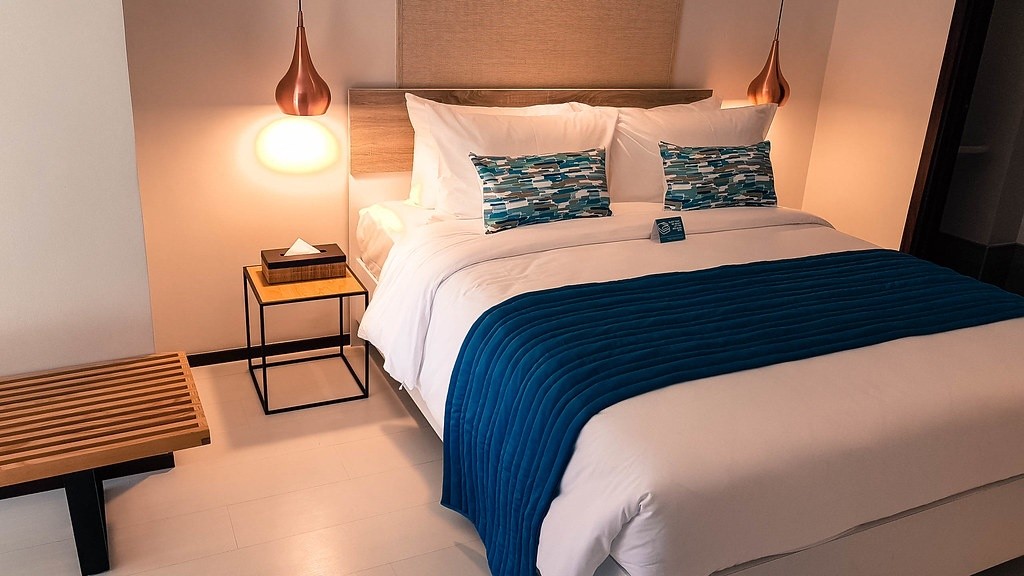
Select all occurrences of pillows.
[647,94,724,111]
[468,145,612,234]
[404,92,593,209]
[426,101,619,218]
[657,140,777,211]
[609,103,779,204]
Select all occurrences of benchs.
[0,351,212,576]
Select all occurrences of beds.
[348,88,1023,576]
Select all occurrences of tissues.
[260,236,347,284]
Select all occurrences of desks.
[243,263,369,414]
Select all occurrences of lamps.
[275,0,332,116]
[746,0,790,107]
